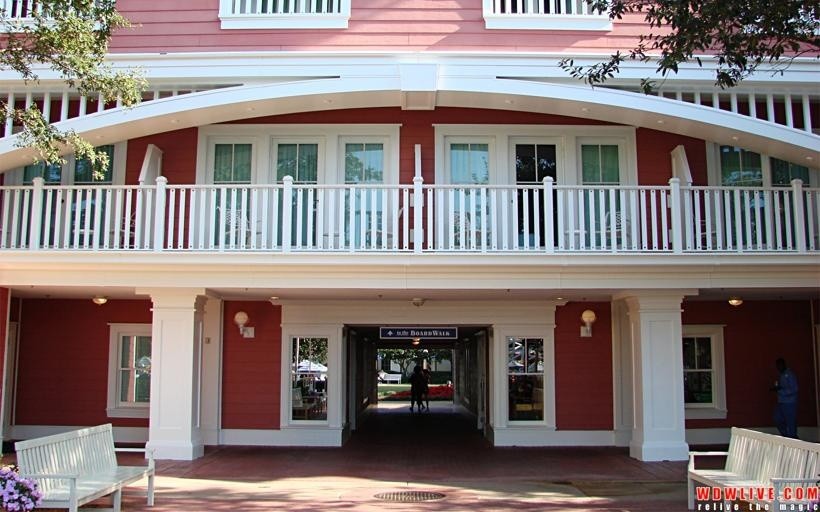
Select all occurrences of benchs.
[291,387,323,420]
[13,422,157,511]
[686,426,819,512]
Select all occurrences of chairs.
[454,210,492,250]
[217,206,263,249]
[367,206,404,249]
[109,210,136,249]
[596,211,632,249]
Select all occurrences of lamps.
[580,310,597,338]
[233,310,255,339]
[728,299,743,306]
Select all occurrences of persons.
[766,355,800,440]
[407,363,431,412]
